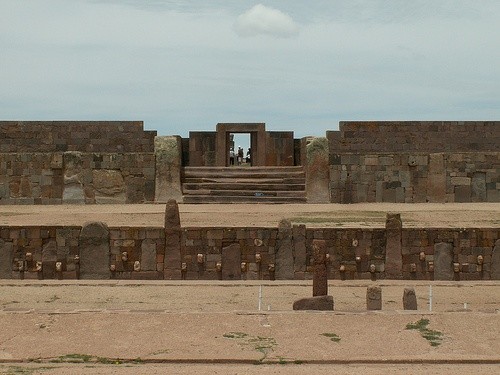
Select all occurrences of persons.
[236,153,242,166]
[229,147,235,165]
[238,147,243,158]
[245,148,251,166]
